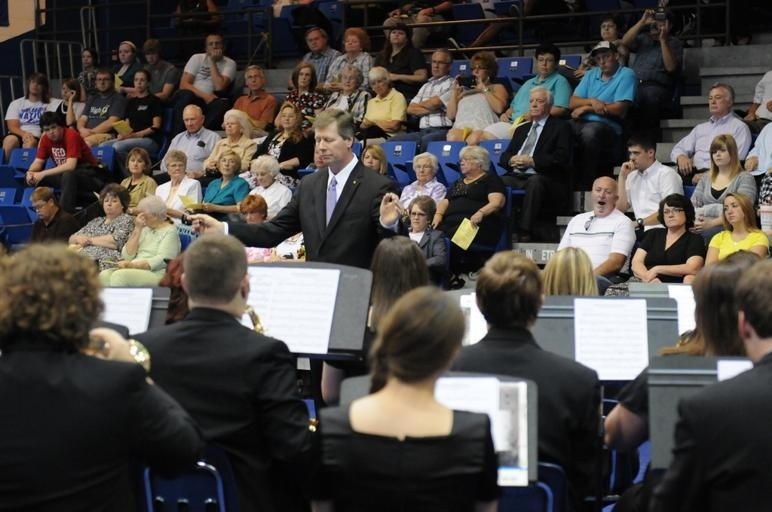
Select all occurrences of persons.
[306,284,499,512]
[604,259,748,453]
[128,231,313,512]
[649,256,772,512]
[0,242,202,512]
[320,235,433,405]
[2,1,771,300]
[448,250,613,512]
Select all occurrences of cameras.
[458,75,476,86]
[653,6,667,20]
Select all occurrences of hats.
[591,40,619,58]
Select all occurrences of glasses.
[411,212,429,218]
[33,203,48,210]
[663,207,685,215]
[431,60,449,66]
[220,158,237,166]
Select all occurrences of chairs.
[9,147,36,181]
[452,3,488,41]
[536,460,583,507]
[380,142,417,192]
[499,188,512,252]
[448,60,470,78]
[21,188,54,224]
[476,139,512,180]
[158,109,174,155]
[297,144,362,176]
[222,1,348,58]
[1,205,34,244]
[426,139,467,188]
[557,54,581,73]
[497,57,533,91]
[90,144,115,171]
[146,465,230,510]
[0,188,16,206]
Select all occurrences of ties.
[325,176,338,228]
